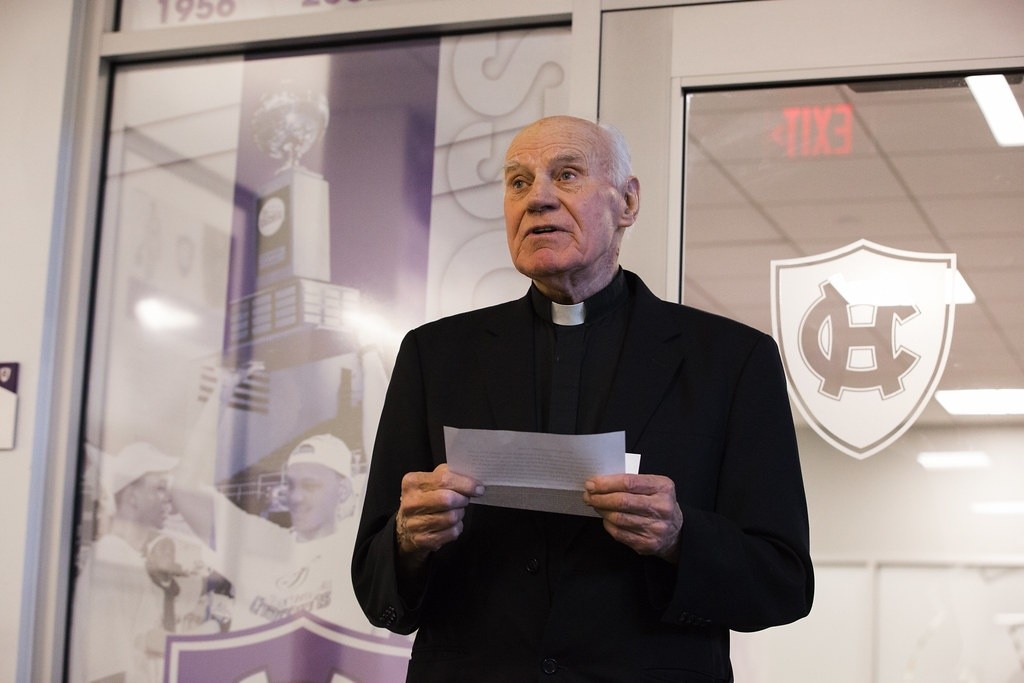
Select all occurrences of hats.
[112,443,182,495]
[289,435,356,516]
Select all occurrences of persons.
[69,441,185,683]
[172,360,391,636]
[350,114,816,683]
